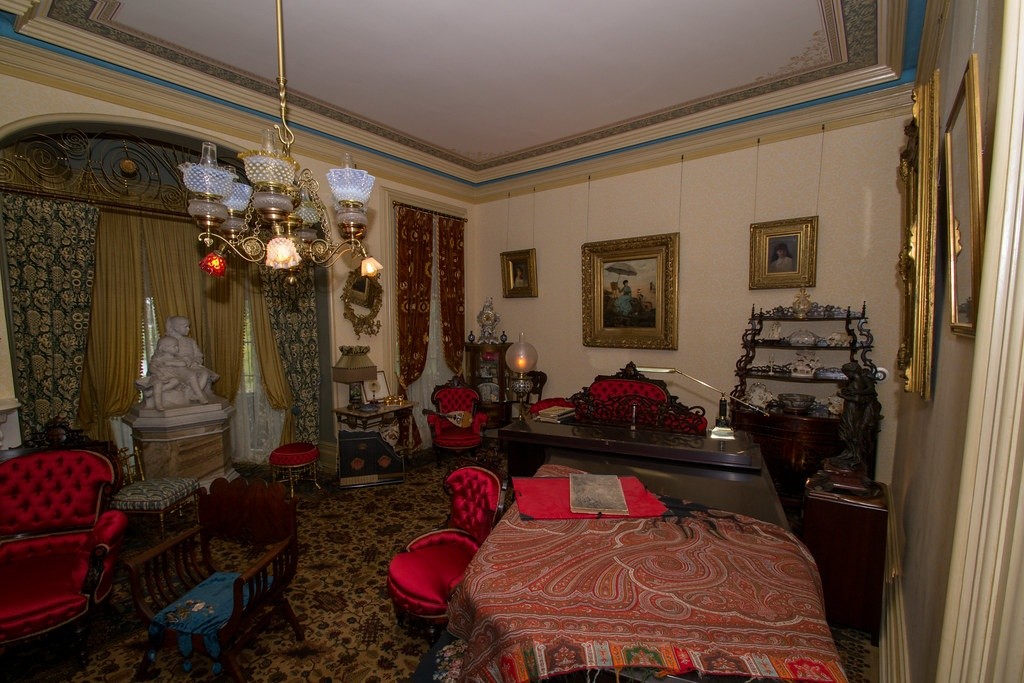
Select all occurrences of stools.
[272,443,322,501]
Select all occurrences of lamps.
[333,344,377,411]
[636,366,772,428]
[510,335,537,424]
[185,0,382,279]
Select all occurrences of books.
[537,406,575,423]
[570,472,629,514]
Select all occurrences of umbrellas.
[605,262,637,286]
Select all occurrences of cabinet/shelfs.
[724,302,887,510]
[792,470,890,615]
[462,341,513,428]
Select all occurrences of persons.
[614,280,632,315]
[514,267,529,287]
[134,315,220,411]
[767,244,796,272]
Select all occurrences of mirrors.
[338,264,384,339]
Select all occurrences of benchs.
[530,362,710,434]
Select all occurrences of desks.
[450,448,847,683]
[495,422,761,490]
[338,397,416,462]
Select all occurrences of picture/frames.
[579,231,679,350]
[749,217,819,289]
[896,56,994,401]
[362,371,390,403]
[500,248,539,299]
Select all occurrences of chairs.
[387,458,501,640]
[0,424,129,659]
[114,447,202,543]
[422,376,488,470]
[122,477,303,682]
